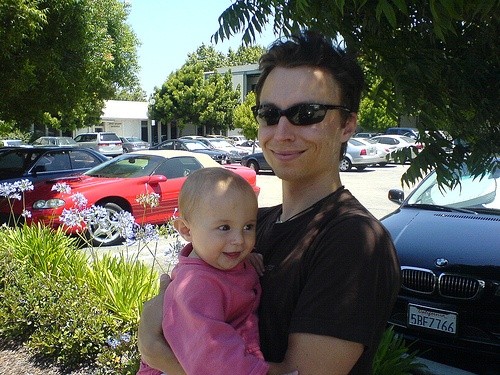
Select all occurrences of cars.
[0,146,144,199]
[377,155,500,357]
[0,127,455,175]
[0,149,261,247]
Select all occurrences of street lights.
[150,98,156,147]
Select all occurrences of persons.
[138,41,401,375]
[136,167,298,375]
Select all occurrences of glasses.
[251,102,351,127]
